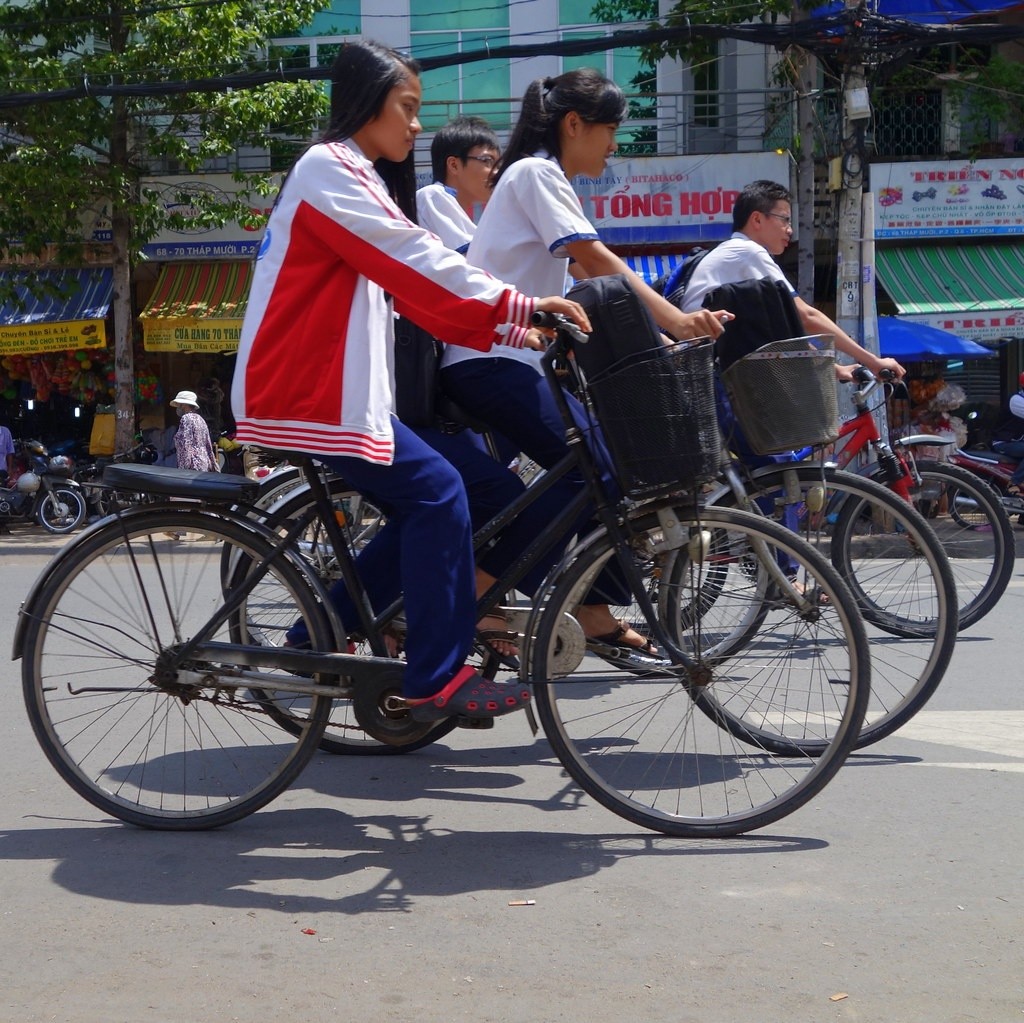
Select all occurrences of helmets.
[17,472,41,495]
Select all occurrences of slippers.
[406,664,532,723]
[277,635,356,684]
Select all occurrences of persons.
[1009,371,1024,527]
[413,112,501,356]
[163,390,221,540]
[0,422,15,535]
[232,41,592,720]
[670,180,905,604]
[434,68,738,666]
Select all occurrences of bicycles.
[8,310,1017,839]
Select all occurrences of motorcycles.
[83,439,171,519]
[946,444,1024,529]
[0,400,87,534]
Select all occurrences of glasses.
[765,212,793,226]
[467,155,502,170]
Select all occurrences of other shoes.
[819,594,833,606]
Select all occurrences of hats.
[1018,373,1024,386]
[169,390,202,411]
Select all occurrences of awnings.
[137,258,256,352]
[0,263,114,356]
[875,241,1024,339]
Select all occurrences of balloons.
[0,335,164,402]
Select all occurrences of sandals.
[592,618,666,661]
[476,606,523,668]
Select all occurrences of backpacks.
[651,237,743,308]
[565,273,704,498]
[701,275,827,450]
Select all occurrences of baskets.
[48,457,74,477]
[1006,480,1020,495]
[586,333,721,497]
[719,332,838,458]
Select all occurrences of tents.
[812,315,999,363]
